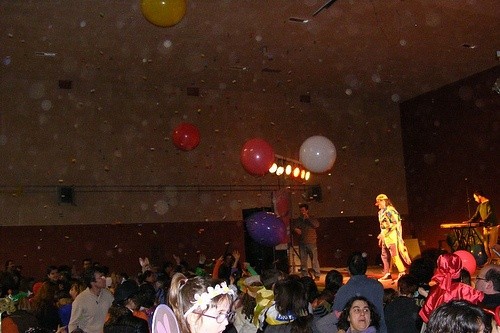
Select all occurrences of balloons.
[467,245,488,266]
[173,123,201,151]
[240,139,274,176]
[246,212,286,247]
[299,136,336,173]
[454,251,476,275]
[141,0,186,27]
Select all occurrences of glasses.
[193,312,234,323]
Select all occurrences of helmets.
[454,249,477,279]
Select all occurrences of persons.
[462,189,500,264]
[375,194,411,281]
[0,253,235,333]
[383,248,500,333]
[293,204,320,281]
[230,250,388,333]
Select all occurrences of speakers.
[57,187,76,207]
[309,185,324,202]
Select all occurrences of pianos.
[439,222,482,250]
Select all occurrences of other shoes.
[313,276,320,281]
[379,275,391,281]
